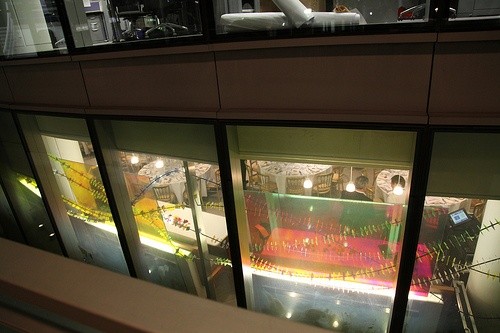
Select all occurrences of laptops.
[447,208,472,227]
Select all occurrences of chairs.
[118,149,487,223]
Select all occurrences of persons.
[339,175,377,235]
[385,174,406,205]
